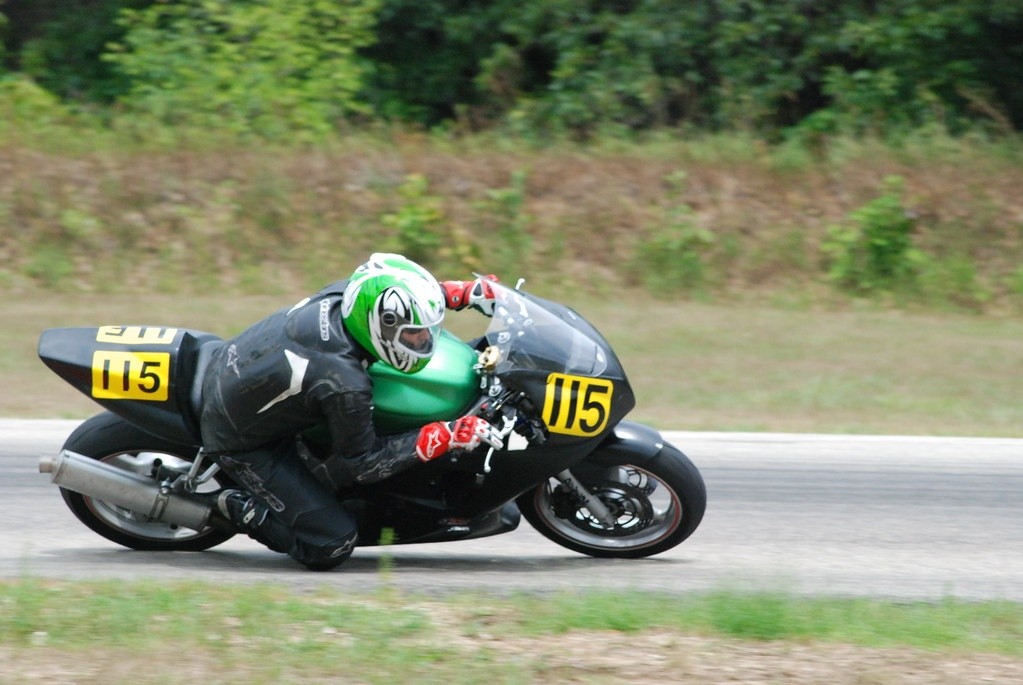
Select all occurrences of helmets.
[341,251,445,374]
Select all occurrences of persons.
[201,252,505,571]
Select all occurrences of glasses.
[401,328,423,334]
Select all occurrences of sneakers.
[217,489,268,531]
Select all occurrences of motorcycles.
[34,269,710,561]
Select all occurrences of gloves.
[415,414,503,462]
[438,274,504,318]
